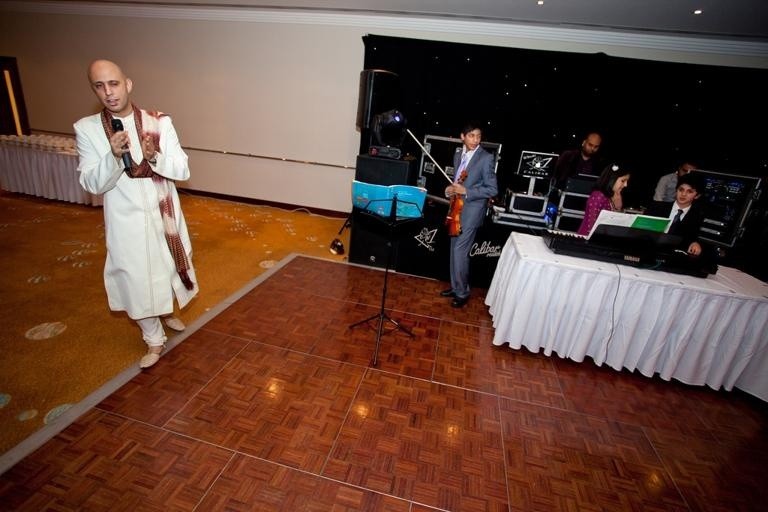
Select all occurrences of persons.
[550,131,603,207]
[640,173,704,256]
[440,126,498,308]
[72,59,200,369]
[652,159,698,204]
[576,163,630,236]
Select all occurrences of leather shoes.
[440,290,457,297]
[163,315,186,332]
[450,295,469,309]
[138,345,164,369]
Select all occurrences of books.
[351,178,428,220]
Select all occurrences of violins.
[444,170,468,238]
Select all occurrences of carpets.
[0,179,356,480]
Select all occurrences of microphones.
[111,118,131,170]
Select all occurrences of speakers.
[356,69,398,129]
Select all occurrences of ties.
[454,156,467,183]
[672,210,683,224]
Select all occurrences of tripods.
[348,195,416,366]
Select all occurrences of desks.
[0,137,104,210]
[480,228,768,405]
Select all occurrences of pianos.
[544,226,717,279]
